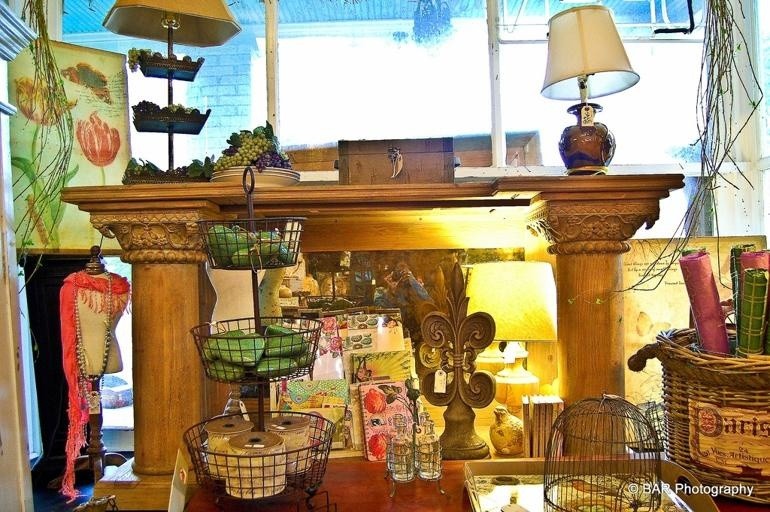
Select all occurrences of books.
[276,308,419,464]
[521,393,564,459]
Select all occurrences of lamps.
[466,261,558,414]
[541,6,641,174]
[102,0,242,183]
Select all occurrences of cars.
[95,369,133,414]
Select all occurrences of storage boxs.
[334,137,455,184]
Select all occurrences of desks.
[182,453,639,511]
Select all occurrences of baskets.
[656,319,770,508]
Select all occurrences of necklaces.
[72,272,112,382]
[81,377,104,409]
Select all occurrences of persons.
[375,256,428,309]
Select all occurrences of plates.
[209,166,304,189]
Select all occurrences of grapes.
[131,100,193,115]
[124,156,214,178]
[128,47,151,72]
[212,120,293,172]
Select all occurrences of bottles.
[384,412,441,481]
[205,418,255,481]
[265,416,315,476]
[224,431,286,499]
